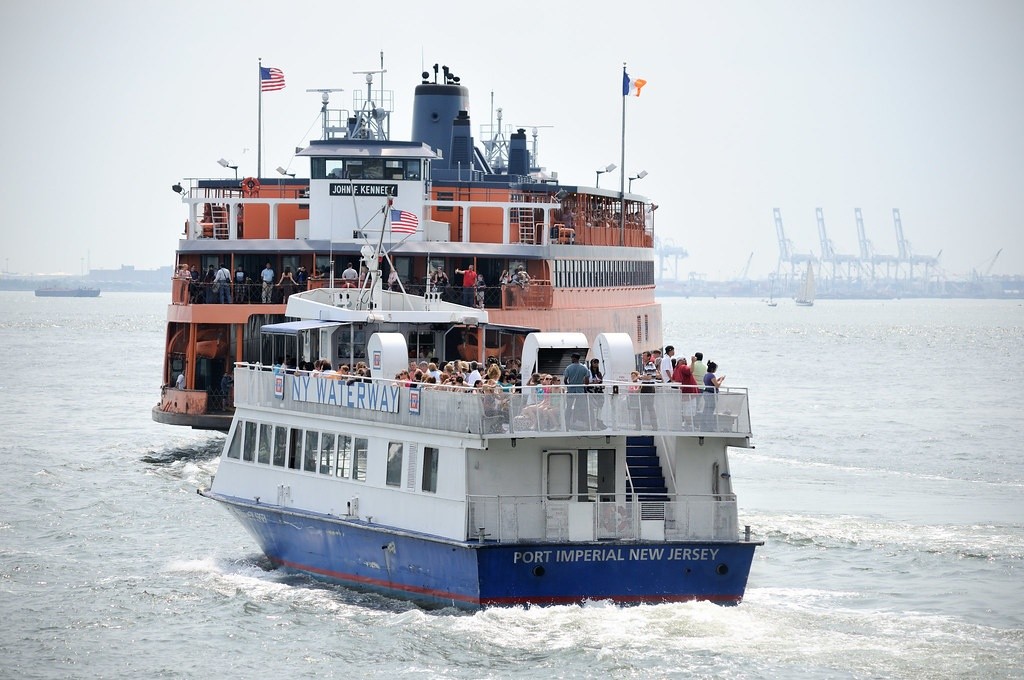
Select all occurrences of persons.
[273,355,372,387]
[393,355,603,432]
[554,206,644,230]
[309,263,322,278]
[201,203,242,238]
[174,370,186,389]
[386,265,397,291]
[292,264,308,292]
[260,262,274,304]
[275,267,298,304]
[627,346,726,431]
[474,274,487,310]
[499,264,536,303]
[233,264,248,304]
[454,264,476,306]
[429,266,449,298]
[341,263,358,288]
[221,370,235,412]
[175,262,232,304]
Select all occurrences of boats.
[767,303,778,307]
[197,284,766,616]
[34,287,101,296]
[151,59,667,433]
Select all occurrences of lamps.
[372,107,386,141]
[629,171,647,194]
[633,203,659,213]
[596,164,615,188]
[216,158,237,181]
[172,182,188,199]
[276,167,296,178]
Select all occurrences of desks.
[694,411,739,434]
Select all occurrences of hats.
[471,361,478,368]
[664,345,674,353]
[590,358,599,363]
[570,353,581,359]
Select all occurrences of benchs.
[546,408,573,431]
[478,394,505,435]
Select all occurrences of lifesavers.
[242,177,260,195]
[603,505,627,532]
[341,282,356,288]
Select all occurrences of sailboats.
[795,260,816,307]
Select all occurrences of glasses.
[540,378,544,380]
[593,361,598,364]
[546,378,551,380]
[556,379,560,382]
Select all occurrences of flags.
[623,72,647,97]
[390,208,418,235]
[260,67,286,92]
